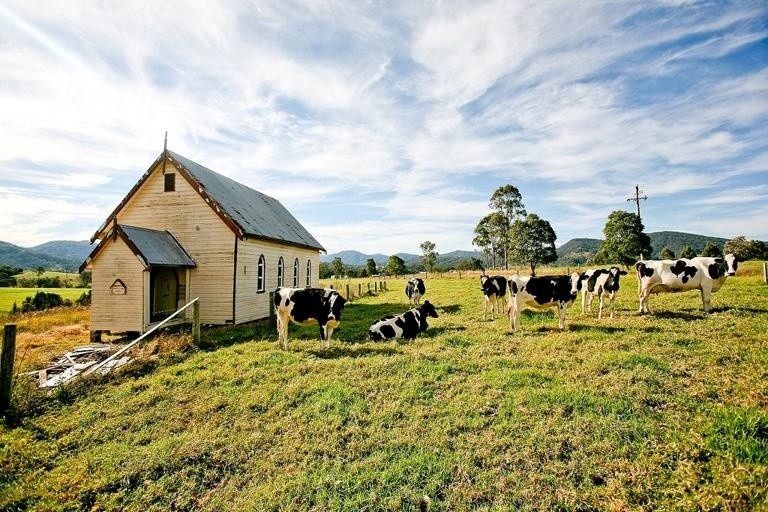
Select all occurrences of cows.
[581,266,628,319]
[480,275,507,322]
[405,277,425,310]
[273,286,346,351]
[635,253,746,316]
[368,300,439,343]
[507,271,590,332]
